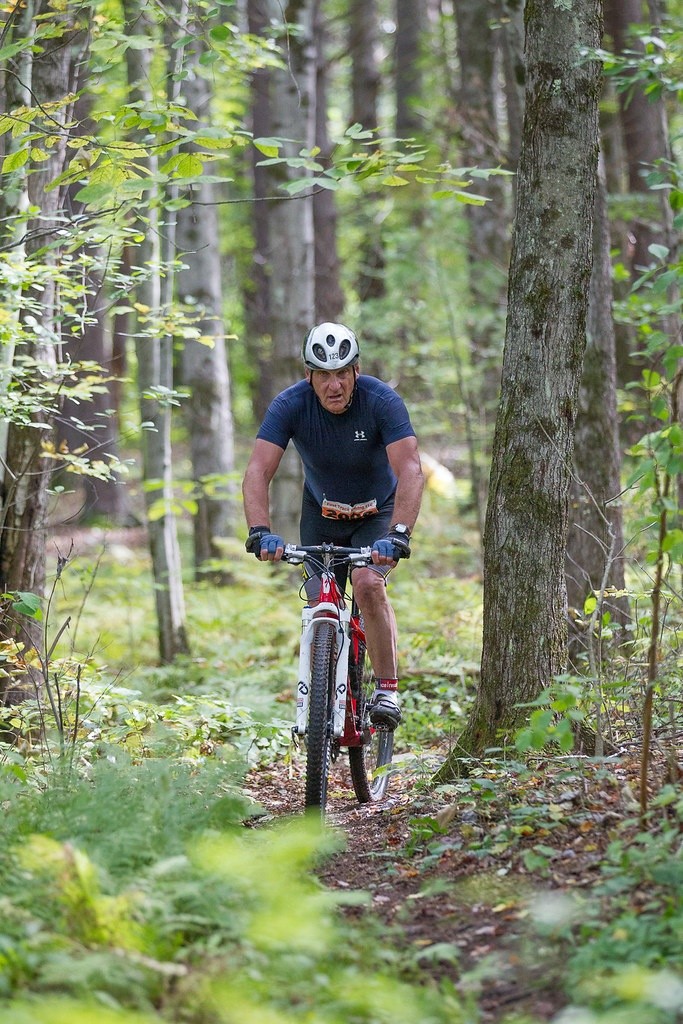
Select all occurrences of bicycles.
[244,531,411,823]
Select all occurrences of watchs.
[390,522,412,536]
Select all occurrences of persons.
[241,321,424,723]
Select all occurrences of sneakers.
[369,693,402,733]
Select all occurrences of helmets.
[300,321,359,371]
